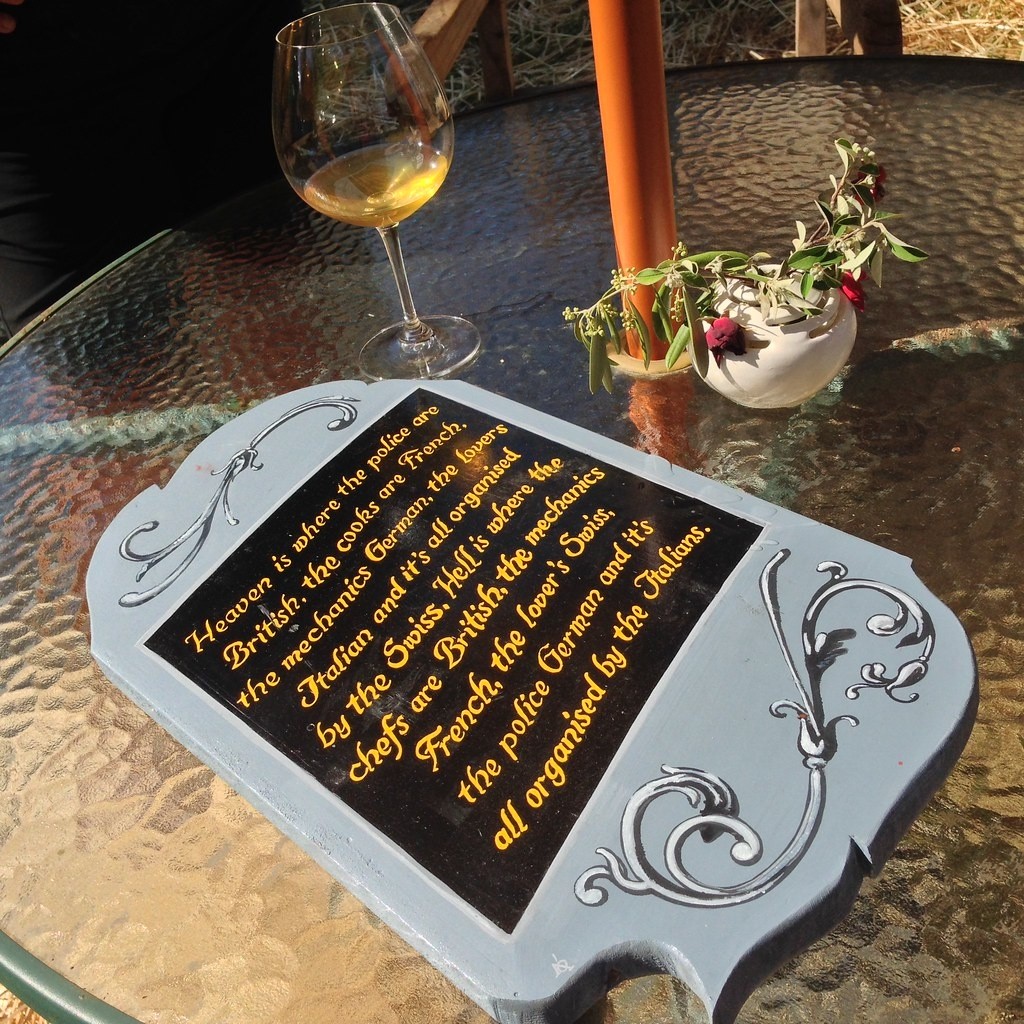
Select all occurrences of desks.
[0,54,1024,1024]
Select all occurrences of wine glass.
[269,2,480,379]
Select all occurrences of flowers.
[560,138,929,395]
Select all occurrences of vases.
[688,270,857,410]
